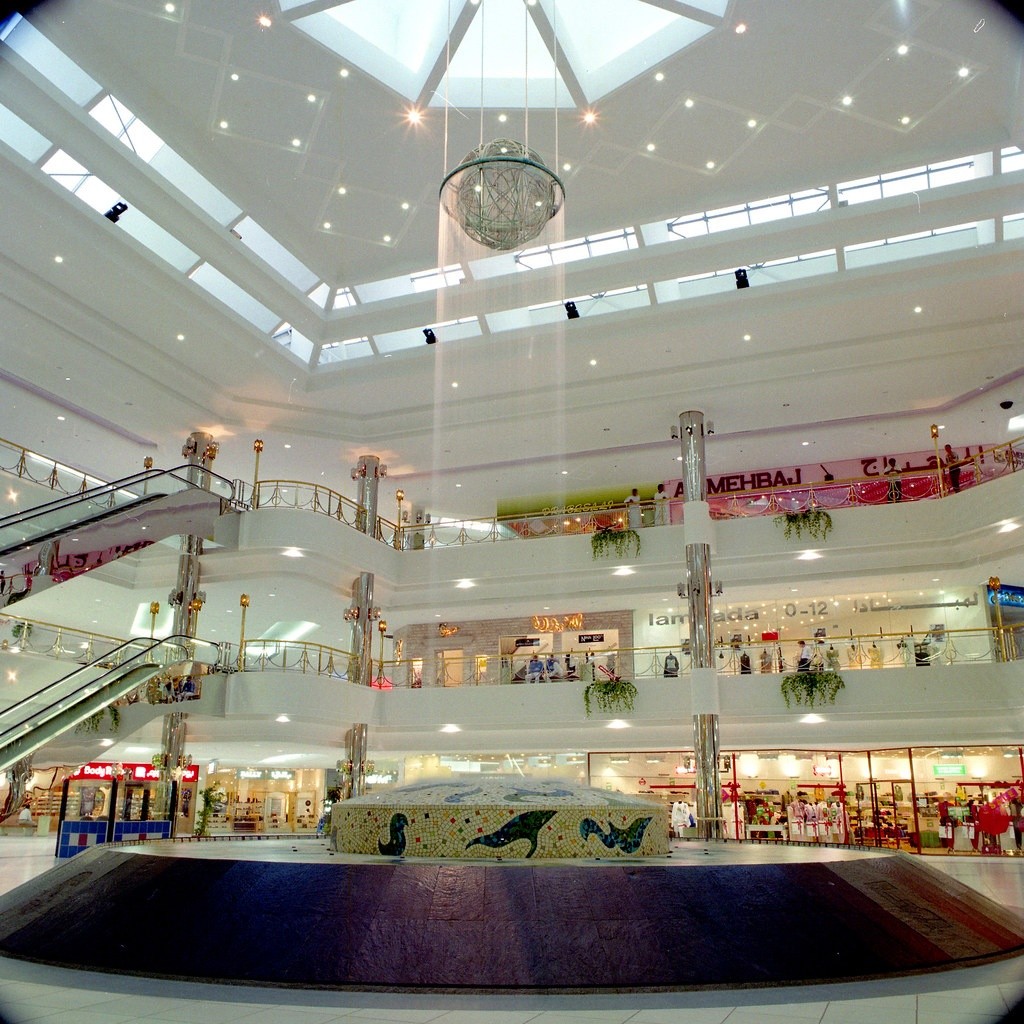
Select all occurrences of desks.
[746,825,786,839]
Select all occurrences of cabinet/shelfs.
[625,793,689,837]
[31,792,156,816]
[233,801,263,833]
[914,796,982,817]
[296,817,318,829]
[207,813,232,833]
[267,817,281,828]
[741,795,916,833]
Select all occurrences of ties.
[800,650,803,659]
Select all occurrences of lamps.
[168,587,183,608]
[677,582,690,599]
[710,579,723,597]
[684,756,697,773]
[368,606,381,621]
[343,606,359,623]
[402,510,410,523]
[701,418,714,438]
[362,759,374,776]
[683,417,693,436]
[415,512,422,523]
[692,576,700,596]
[351,461,366,481]
[336,759,352,775]
[193,591,206,604]
[177,754,192,770]
[152,752,168,770]
[207,440,220,461]
[717,754,731,773]
[670,425,682,439]
[375,464,387,480]
[181,437,197,459]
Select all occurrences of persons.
[545,647,616,682]
[796,641,813,671]
[165,676,195,703]
[653,484,671,526]
[663,638,912,678]
[624,489,642,527]
[884,458,903,504]
[19,804,37,827]
[525,655,544,683]
[944,445,961,493]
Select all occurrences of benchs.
[175,695,201,702]
[0,823,38,836]
